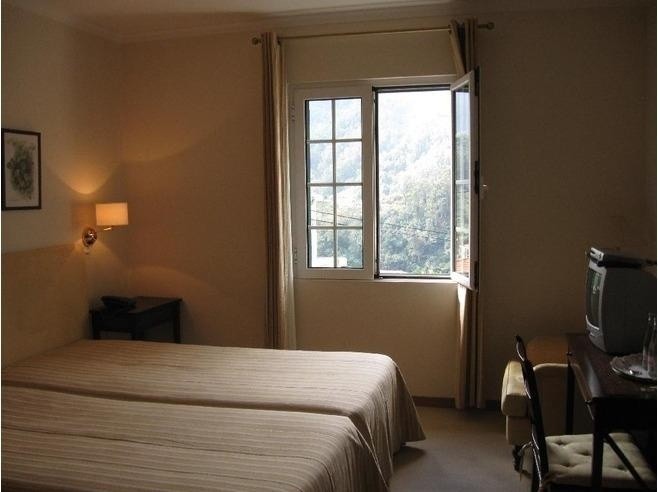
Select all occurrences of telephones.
[101,296,136,311]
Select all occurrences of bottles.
[640,312,656,377]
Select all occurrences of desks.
[91,296,183,343]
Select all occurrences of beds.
[1,243,408,489]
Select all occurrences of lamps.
[81,200,129,248]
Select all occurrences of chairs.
[501,334,656,491]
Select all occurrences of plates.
[610,352,657,385]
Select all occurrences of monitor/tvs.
[585,258,657,356]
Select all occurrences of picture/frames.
[1,127,42,211]
